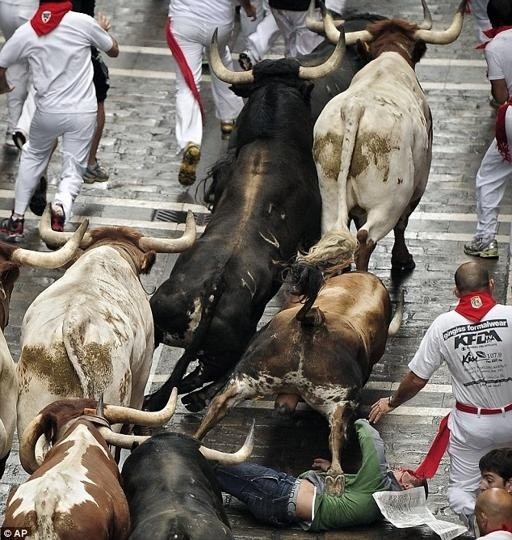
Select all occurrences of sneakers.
[11,128,27,150]
[220,119,236,142]
[462,238,500,260]
[177,141,201,188]
[0,210,27,240]
[50,202,66,233]
[238,46,262,71]
[30,176,48,218]
[83,158,112,184]
[4,130,16,147]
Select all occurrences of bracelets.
[388,396,398,408]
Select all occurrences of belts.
[454,400,512,418]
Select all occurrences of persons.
[463,0,512,258]
[469,0,492,43]
[219,417,428,535]
[472,486,512,540]
[479,447,512,495]
[367,260,512,532]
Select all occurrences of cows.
[96,392,256,540]
[0,218,89,480]
[0,386,178,540]
[193,227,405,498]
[194,0,433,214]
[296,0,466,279]
[16,202,196,464]
[132,25,346,438]
